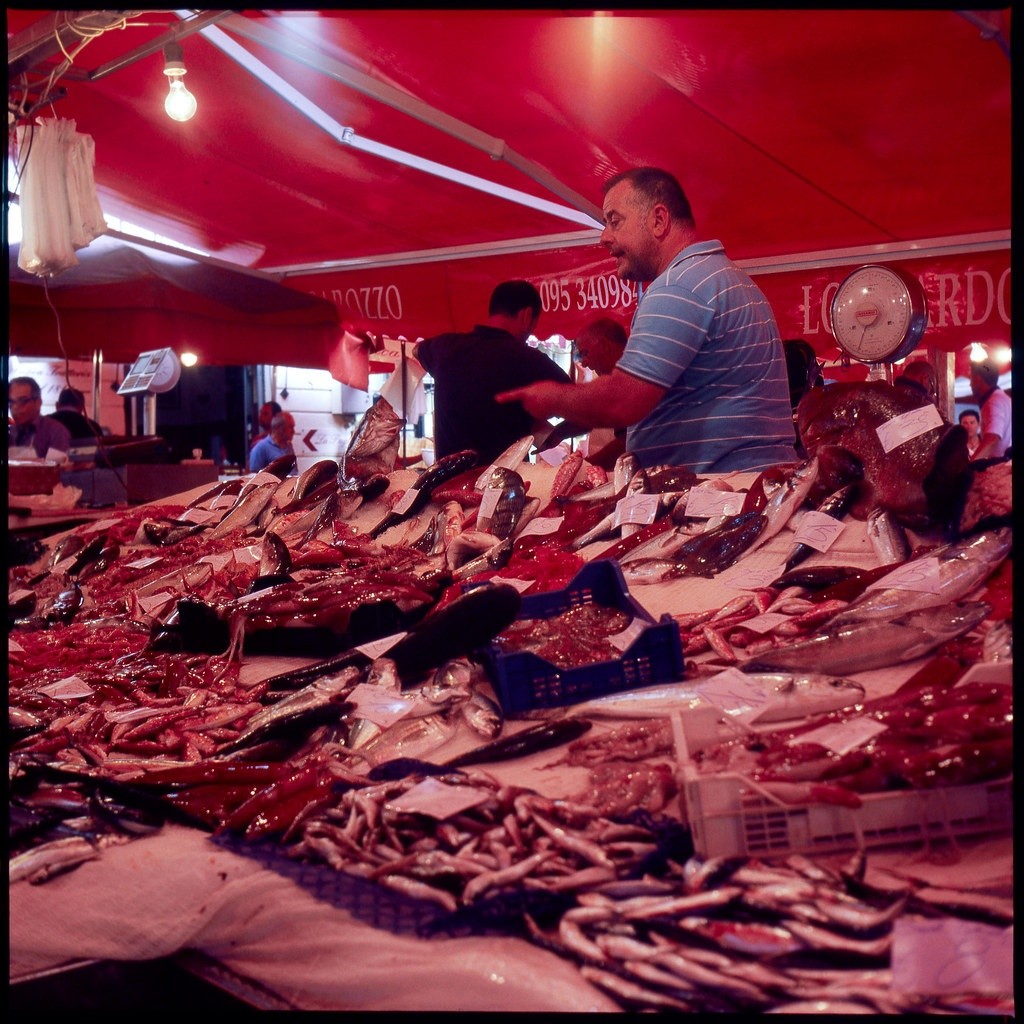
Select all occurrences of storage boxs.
[460,557,682,719]
[667,706,1015,858]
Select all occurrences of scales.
[791,264,928,424]
[67,347,181,467]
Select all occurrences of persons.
[495,168,802,473]
[574,318,628,472]
[46,388,103,439]
[251,401,282,449]
[250,413,299,476]
[8,378,70,463]
[412,278,573,463]
[894,361,937,404]
[959,360,1012,463]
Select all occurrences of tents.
[6,13,1013,470]
[8,232,343,438]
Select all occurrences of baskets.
[207,757,667,941]
[470,559,686,713]
[671,707,1014,862]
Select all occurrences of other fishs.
[295,759,915,1016]
[42,396,1012,801]
[7,618,349,887]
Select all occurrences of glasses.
[8,396,33,407]
[929,380,937,398]
